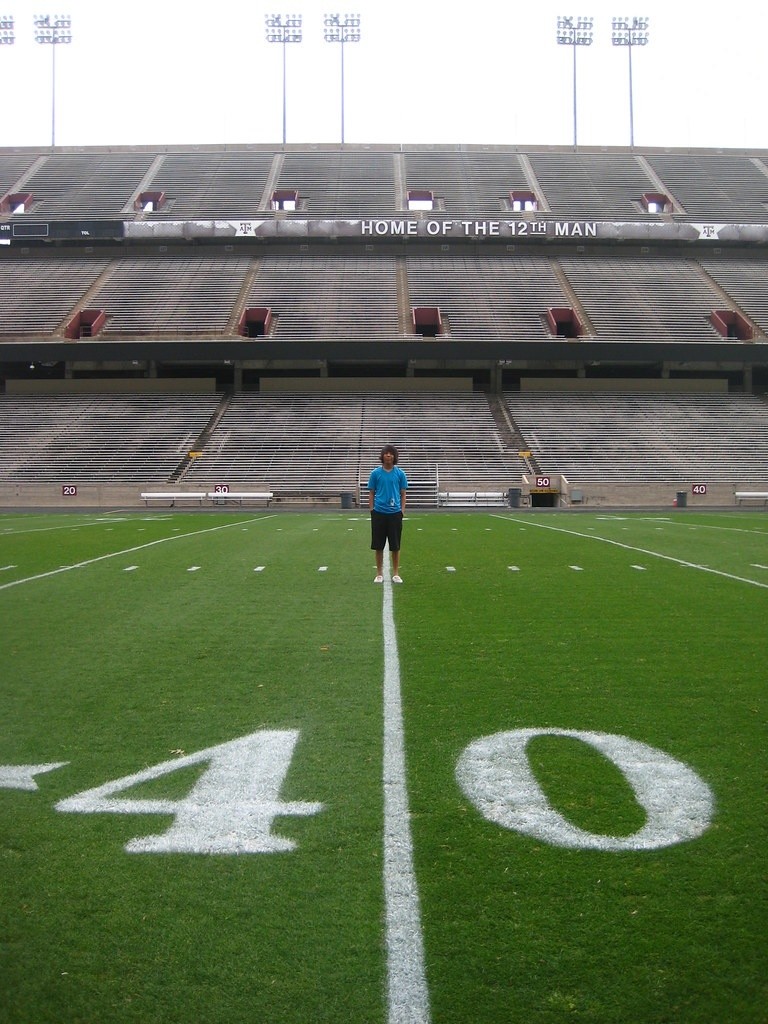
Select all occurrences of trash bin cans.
[676,492,687,507]
[509,487,521,509]
[340,492,353,509]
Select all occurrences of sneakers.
[392,575,403,583]
[373,575,384,583]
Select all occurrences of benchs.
[207,493,273,507]
[437,492,507,506]
[733,492,768,506]
[359,492,439,509]
[139,492,207,506]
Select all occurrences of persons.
[368,445,408,584]
